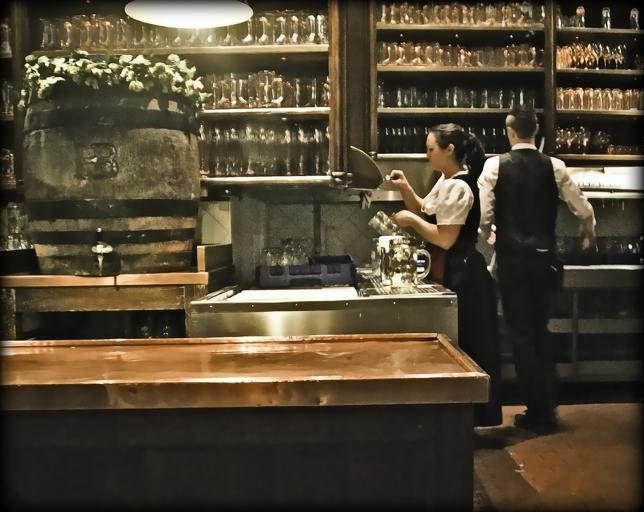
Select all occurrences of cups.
[38,11,330,48]
[374,0,547,108]
[367,209,401,238]
[556,233,644,263]
[195,68,331,178]
[0,21,34,251]
[554,3,644,154]
[264,236,311,276]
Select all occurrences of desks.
[1,329,489,510]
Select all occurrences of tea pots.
[371,233,433,288]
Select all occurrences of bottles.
[376,125,511,156]
[134,312,174,339]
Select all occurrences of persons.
[477,104,594,432]
[391,123,501,427]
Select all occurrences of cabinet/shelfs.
[363,1,552,169]
[0,3,345,208]
[553,0,642,196]
[488,262,640,388]
